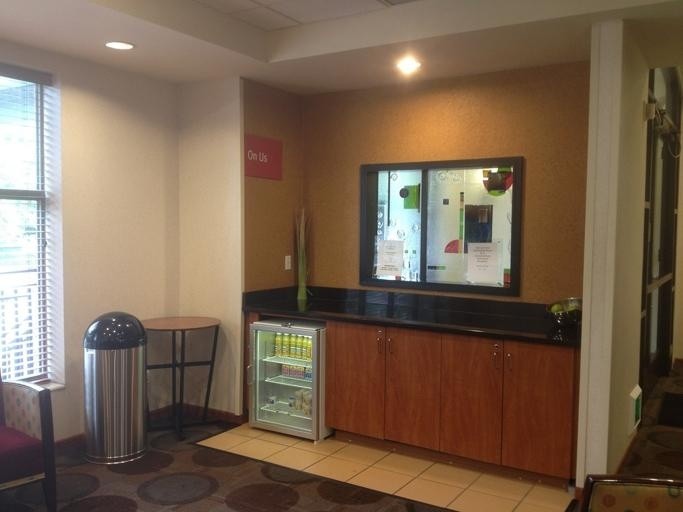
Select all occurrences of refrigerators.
[247,318,334,442]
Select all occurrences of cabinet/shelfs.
[442,333,579,490]
[325,319,442,460]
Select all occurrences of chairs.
[0,371,57,512]
[578,475,683,512]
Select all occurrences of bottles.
[270,329,313,360]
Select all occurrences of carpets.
[0,414,454,512]
[659,391,683,427]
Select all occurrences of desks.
[141,317,221,439]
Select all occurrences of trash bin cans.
[81,313,147,464]
